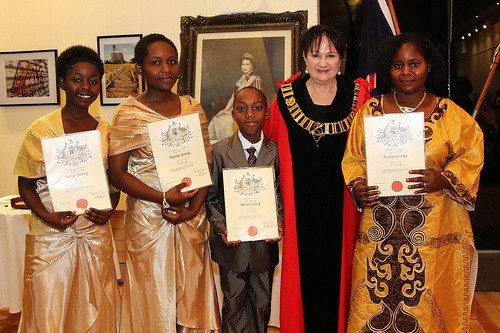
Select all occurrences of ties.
[245,147,258,167]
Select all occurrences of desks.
[0,194,33,313]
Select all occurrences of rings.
[424,183,426,188]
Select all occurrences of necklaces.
[394,90,426,113]
[281,82,360,147]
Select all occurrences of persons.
[15,25,485,332]
[208,53,264,146]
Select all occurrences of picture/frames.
[0,48,60,107]
[177,8,309,144]
[97,34,145,106]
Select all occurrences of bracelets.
[162,191,169,209]
